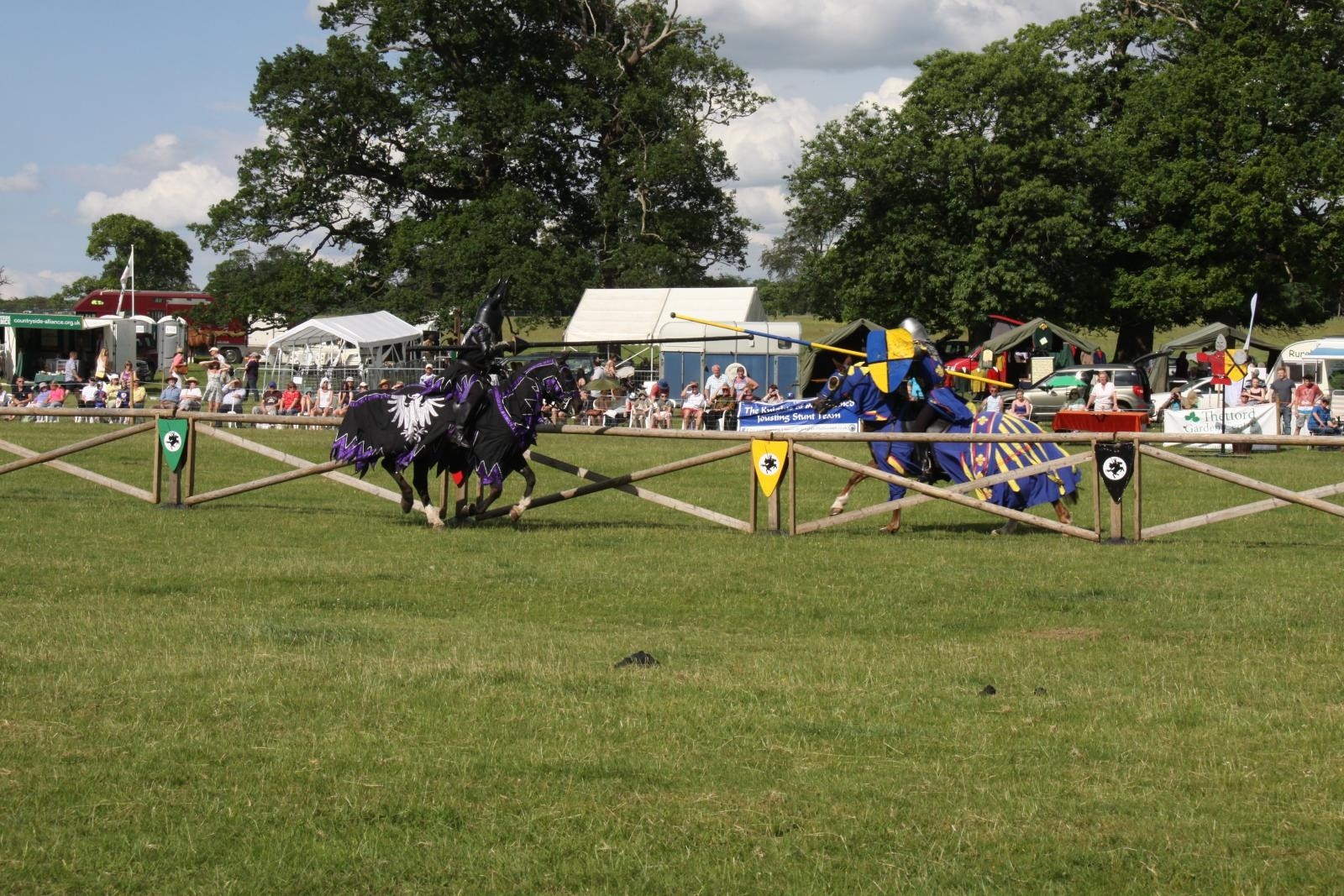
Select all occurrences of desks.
[34,374,64,384]
[1052,410,1151,432]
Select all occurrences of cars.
[1147,375,1269,424]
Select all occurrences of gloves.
[500,341,511,352]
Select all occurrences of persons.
[445,306,509,448]
[955,367,1001,392]
[419,335,439,385]
[510,353,783,431]
[0,346,404,430]
[1093,346,1106,364]
[899,318,974,482]
[977,371,1120,432]
[1158,347,1342,452]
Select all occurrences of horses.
[336,350,582,529]
[812,356,1084,537]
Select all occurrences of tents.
[797,319,889,399]
[977,316,1097,401]
[562,288,768,380]
[1148,322,1286,393]
[262,310,421,398]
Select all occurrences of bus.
[72,289,250,366]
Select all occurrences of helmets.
[899,317,932,343]
[473,273,513,335]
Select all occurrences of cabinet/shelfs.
[56,359,79,374]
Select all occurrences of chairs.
[1027,404,1033,420]
[588,398,729,431]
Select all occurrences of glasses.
[1071,392,1078,395]
[288,385,296,388]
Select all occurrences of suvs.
[942,343,1106,398]
[981,363,1149,425]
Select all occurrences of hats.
[111,374,119,378]
[629,392,639,399]
[657,379,668,390]
[164,375,178,383]
[90,376,96,381]
[269,382,276,388]
[1171,386,1181,393]
[426,364,433,369]
[185,377,198,384]
[638,390,648,398]
[345,377,355,382]
[209,347,219,352]
[1318,396,1330,402]
[39,382,47,389]
[359,381,368,387]
[380,379,389,385]
[1302,374,1312,380]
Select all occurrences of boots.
[450,392,485,448]
[919,441,938,479]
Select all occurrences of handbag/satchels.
[95,371,104,378]
[174,365,188,375]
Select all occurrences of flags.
[120,250,133,290]
